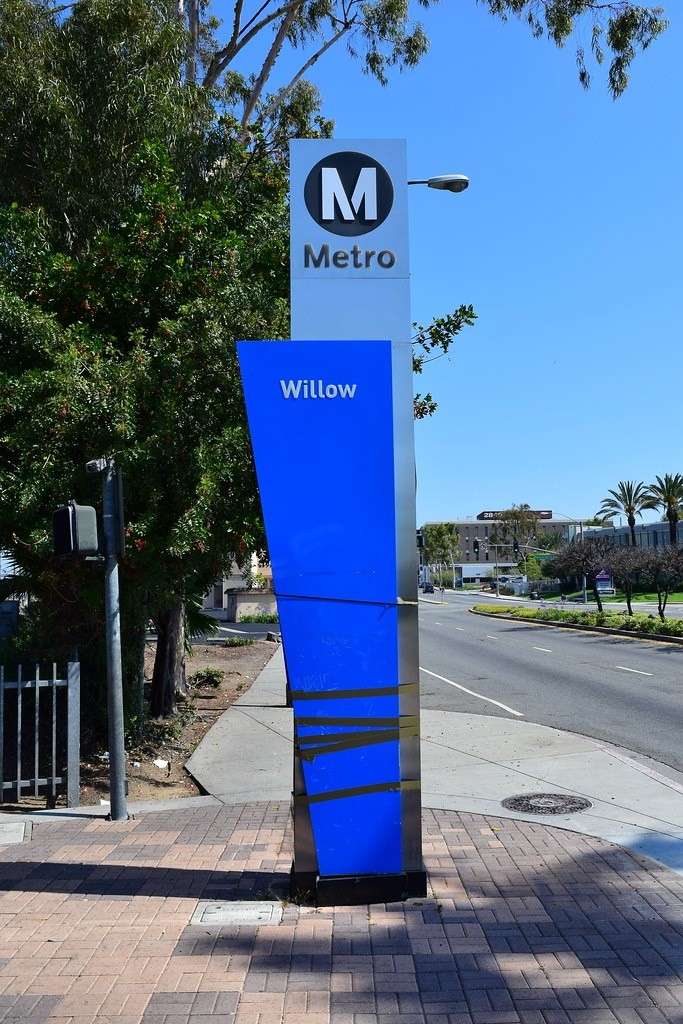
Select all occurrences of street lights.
[540,512,587,604]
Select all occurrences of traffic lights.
[474,541,479,553]
[513,541,518,552]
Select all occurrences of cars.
[512,576,524,583]
[422,585,435,593]
[419,582,431,588]
